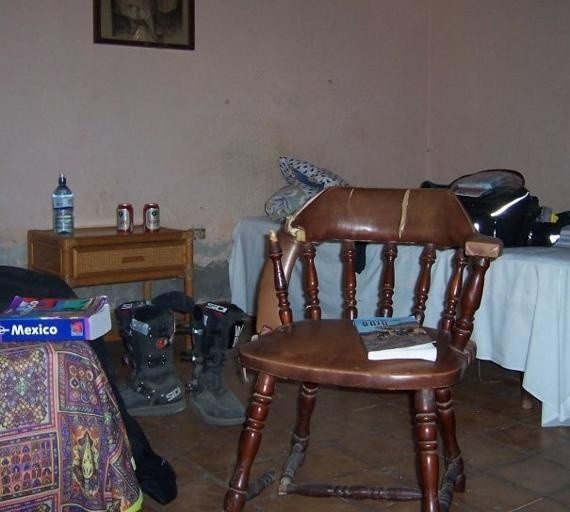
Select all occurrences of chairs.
[223,185,502,511]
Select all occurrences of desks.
[27,226,194,361]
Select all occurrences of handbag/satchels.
[419,168,539,249]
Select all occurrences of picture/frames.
[92,0,195,50]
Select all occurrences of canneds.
[117,203,134,232]
[143,203,160,232]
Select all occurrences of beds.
[1,262,143,512]
[228,215,569,434]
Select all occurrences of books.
[352,315,438,363]
[0,295,112,342]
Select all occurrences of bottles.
[51,174,75,234]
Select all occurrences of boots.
[115,301,186,417]
[189,302,246,426]
